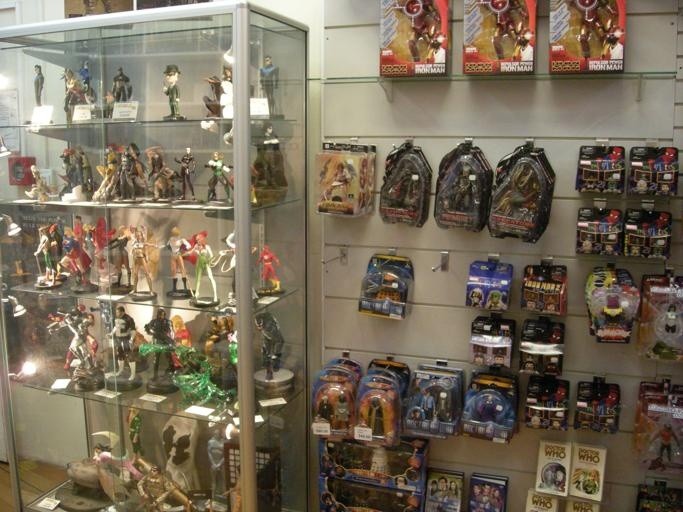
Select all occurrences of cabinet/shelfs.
[0,2,313,510]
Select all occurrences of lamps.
[0,130,36,511]
[218,44,259,511]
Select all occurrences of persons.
[162,226,283,306]
[477,1,529,61]
[5,293,285,418]
[394,0,440,62]
[204,122,288,201]
[163,64,181,117]
[317,163,680,511]
[202,55,279,117]
[34,65,44,106]
[24,144,95,202]
[112,66,133,102]
[1,213,164,296]
[60,61,114,119]
[568,1,618,59]
[92,143,196,201]
[91,406,241,512]
